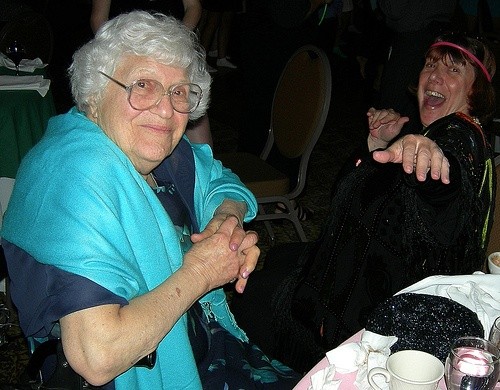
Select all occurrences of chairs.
[208,47,332,242]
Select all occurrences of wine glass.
[488,316,500,382]
[6,39,26,75]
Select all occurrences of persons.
[378,0,456,115]
[91,0,214,151]
[202,0,238,68]
[245,0,394,75]
[459,0,500,32]
[258,33,496,373]
[0,11,305,390]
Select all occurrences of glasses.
[98,70,204,114]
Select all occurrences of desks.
[0,66,71,180]
[285,270,500,390]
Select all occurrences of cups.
[445,336,500,390]
[367,350,445,390]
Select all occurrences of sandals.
[272,198,315,227]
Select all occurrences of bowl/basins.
[488,252,500,274]
